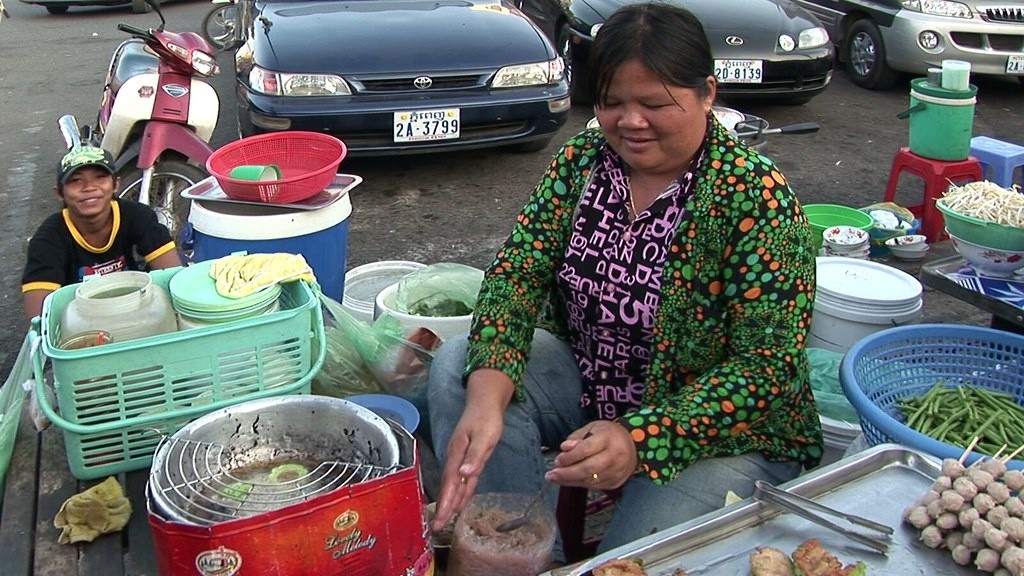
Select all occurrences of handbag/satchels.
[0,321,41,499]
[309,285,441,424]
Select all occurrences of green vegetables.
[408,298,473,316]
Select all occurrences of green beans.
[894,378,1024,462]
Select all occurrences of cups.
[942,60,971,91]
[229,163,281,201]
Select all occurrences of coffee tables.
[969,136,1024,192]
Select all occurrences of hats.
[58,145,116,188]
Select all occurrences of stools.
[885,147,983,245]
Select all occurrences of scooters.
[56,0,245,258]
[200,1,251,51]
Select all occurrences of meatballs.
[902,458,1024,576]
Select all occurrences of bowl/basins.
[885,234,930,262]
[822,226,872,262]
[343,394,422,435]
[149,393,401,526]
[935,197,1024,279]
[858,208,919,258]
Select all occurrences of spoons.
[495,478,551,533]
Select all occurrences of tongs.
[753,479,894,554]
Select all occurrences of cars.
[17,0,165,15]
[232,0,571,158]
[791,0,1024,89]
[512,0,836,106]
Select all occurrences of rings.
[461,476,467,484]
[592,474,600,485]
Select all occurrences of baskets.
[838,323,1024,475]
[206,130,347,205]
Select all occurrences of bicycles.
[587,104,822,157]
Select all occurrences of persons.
[23,146,184,334]
[428,2,824,574]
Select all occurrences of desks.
[919,254,1024,359]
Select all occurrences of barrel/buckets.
[180,193,354,319]
[342,259,475,386]
[807,256,924,356]
[802,203,875,260]
[896,78,980,162]
[61,270,178,416]
